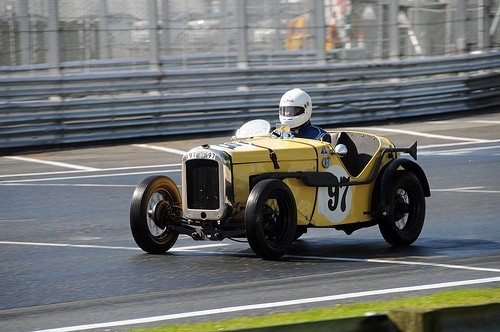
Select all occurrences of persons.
[268,89,332,146]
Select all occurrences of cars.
[130,128,432,263]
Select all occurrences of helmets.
[279,88,312,129]
